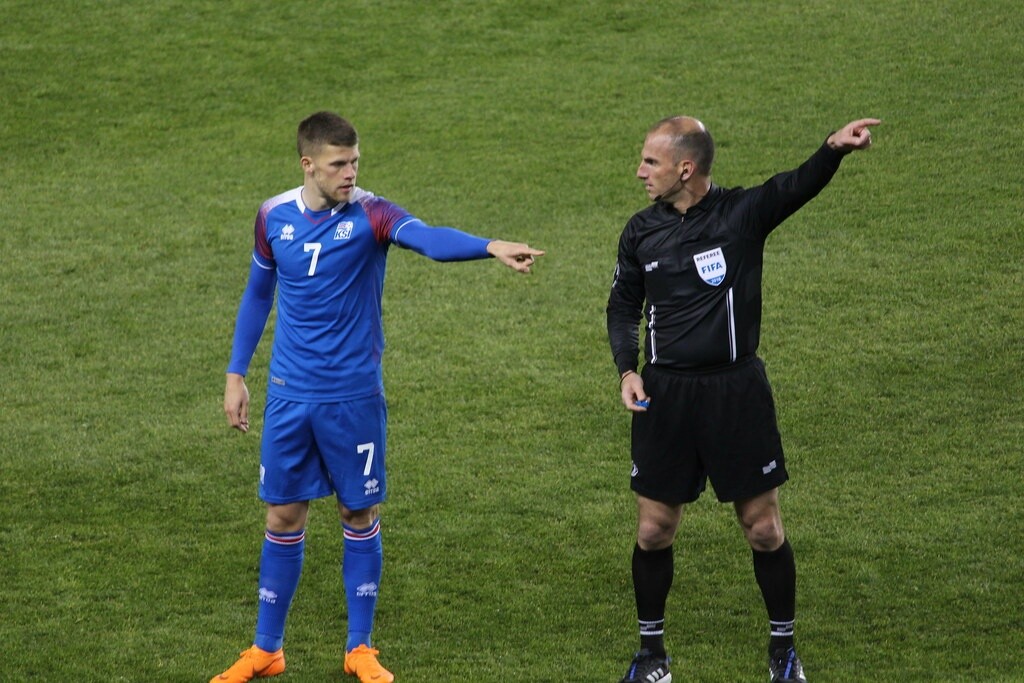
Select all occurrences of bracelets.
[618,370,635,391]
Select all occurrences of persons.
[208,108,549,683]
[605,113,881,683]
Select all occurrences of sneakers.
[621,648,672,683]
[769,647,807,683]
[209,644,285,683]
[344,644,394,683]
